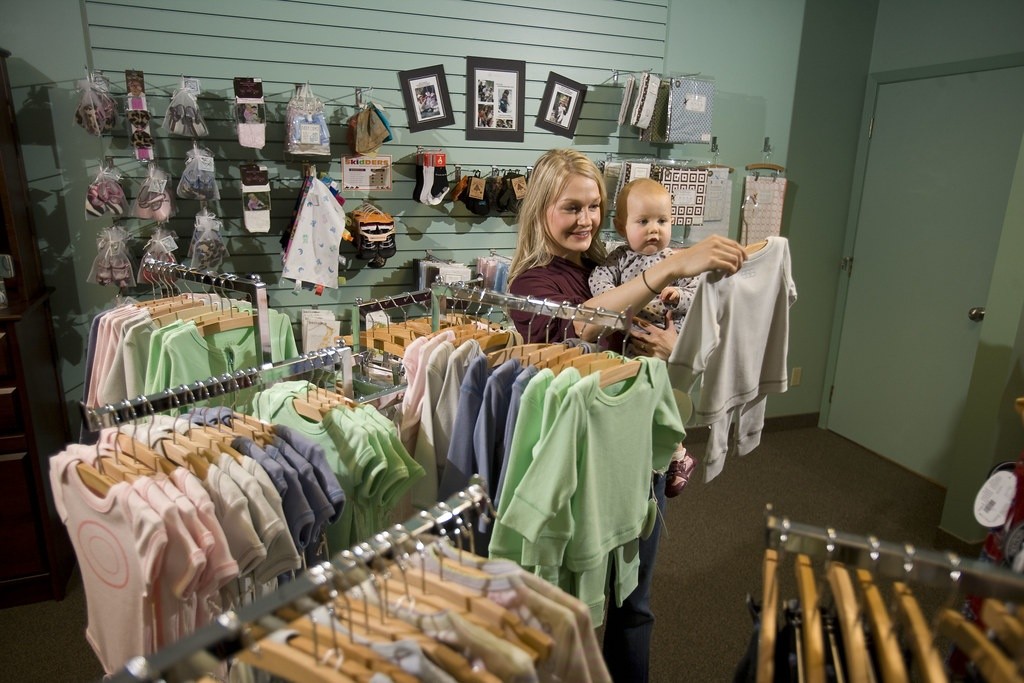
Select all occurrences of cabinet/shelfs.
[0,47,83,611]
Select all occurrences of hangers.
[76,257,641,683]
[736,221,765,256]
[754,507,1024,683]
[744,150,785,182]
[694,146,734,173]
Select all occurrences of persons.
[588,178,703,499]
[478,82,513,128]
[505,147,748,683]
[243,104,258,121]
[248,193,264,211]
[554,95,569,125]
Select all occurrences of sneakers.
[665,452,697,498]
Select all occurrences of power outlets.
[790,368,802,386]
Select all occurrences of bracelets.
[642,270,661,295]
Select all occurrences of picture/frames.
[465,56,526,143]
[396,64,455,135]
[534,71,587,139]
